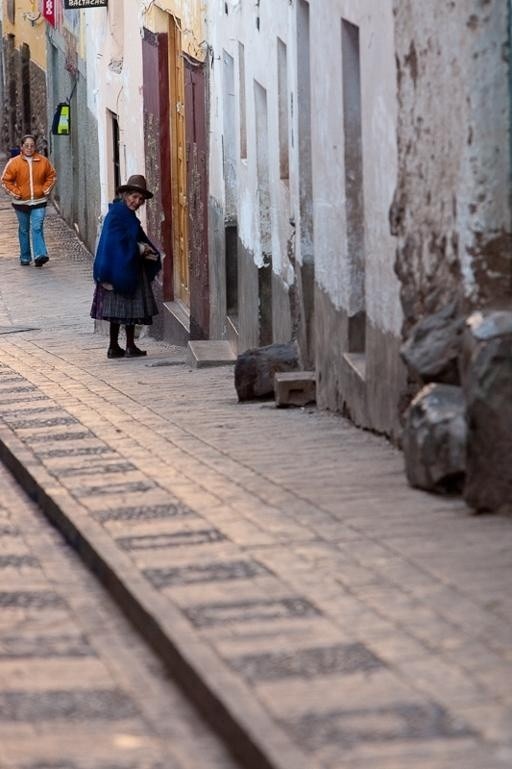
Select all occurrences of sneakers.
[123,346,147,358]
[20,255,49,267]
[106,345,126,359]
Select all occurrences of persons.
[88,173,156,359]
[0,134,56,268]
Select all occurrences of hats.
[115,174,153,199]
